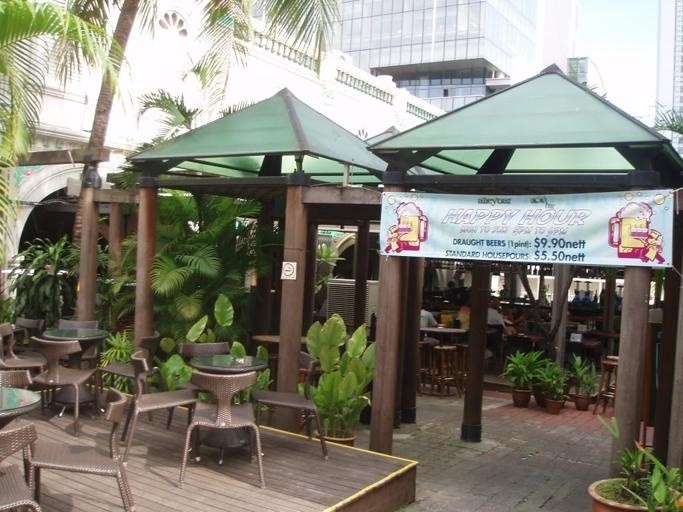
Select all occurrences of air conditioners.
[325,276,379,338]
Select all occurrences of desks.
[427,292,621,374]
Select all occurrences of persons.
[420,281,511,334]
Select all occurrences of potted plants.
[567,351,597,411]
[532,357,574,415]
[297,313,376,447]
[586,414,683,512]
[496,349,554,408]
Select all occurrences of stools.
[418,324,470,399]
[592,355,619,416]
